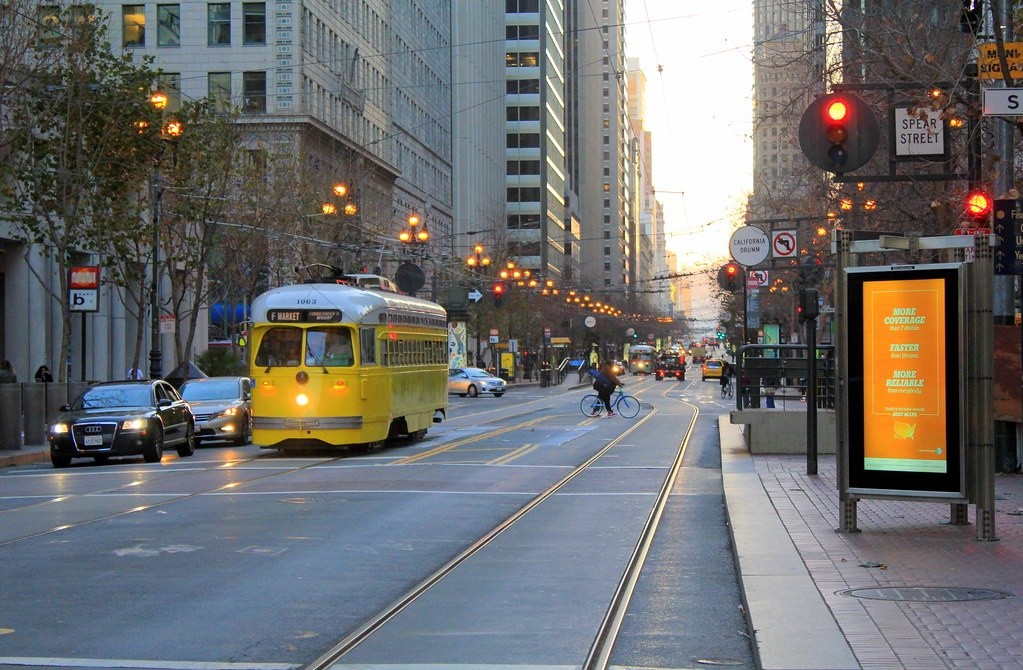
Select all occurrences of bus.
[239,274,449,454]
[630,342,655,376]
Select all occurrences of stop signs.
[747,277,758,289]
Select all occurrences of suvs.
[655,353,687,381]
[701,358,724,381]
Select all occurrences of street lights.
[468,246,490,369]
[501,263,537,341]
[131,93,184,379]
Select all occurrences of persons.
[128,366,144,379]
[35,365,53,383]
[325,332,352,363]
[762,377,780,408]
[0,360,17,383]
[720,362,735,393]
[590,359,622,417]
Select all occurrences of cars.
[48,379,195,468]
[671,347,679,353]
[448,367,507,398]
[177,376,252,444]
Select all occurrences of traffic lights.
[494,283,503,306]
[798,293,806,323]
[728,265,737,291]
[964,190,991,219]
[825,98,849,165]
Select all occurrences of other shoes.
[607,412,618,417]
[591,412,601,416]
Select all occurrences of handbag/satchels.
[593,374,616,395]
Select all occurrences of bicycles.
[721,376,734,400]
[580,383,640,418]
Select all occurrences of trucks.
[692,347,705,363]
